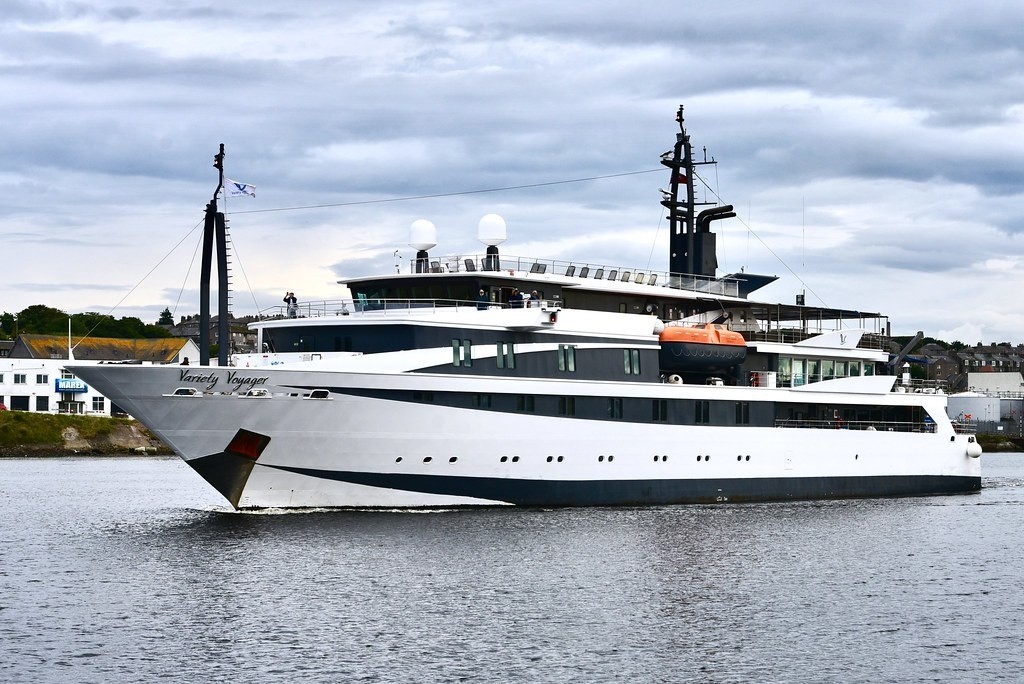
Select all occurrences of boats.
[62,103,982,511]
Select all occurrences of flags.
[224,178,256,199]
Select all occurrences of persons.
[283,292,298,317]
[477,289,489,311]
[526,291,542,309]
[866,423,877,431]
[510,287,524,309]
[180,357,190,366]
